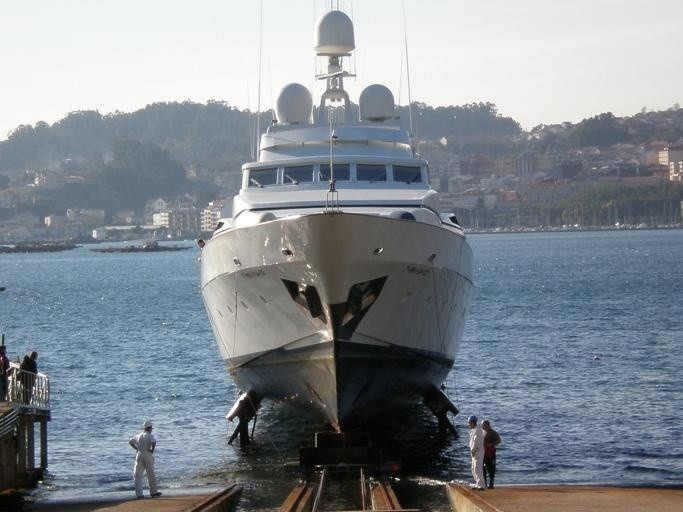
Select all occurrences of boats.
[58,230,102,244]
[0,240,83,253]
[88,238,195,253]
[196,0,475,442]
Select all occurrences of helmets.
[142,421,153,428]
[467,416,476,423]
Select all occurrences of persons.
[467,415,487,491]
[480,419,501,488]
[128,422,162,498]
[0,345,9,401]
[16,351,38,404]
